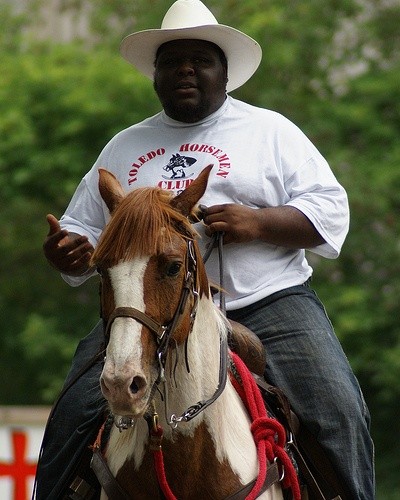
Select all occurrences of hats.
[121,0,263,93]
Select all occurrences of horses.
[85,164,310,500]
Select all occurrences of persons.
[31,1,379,499]
